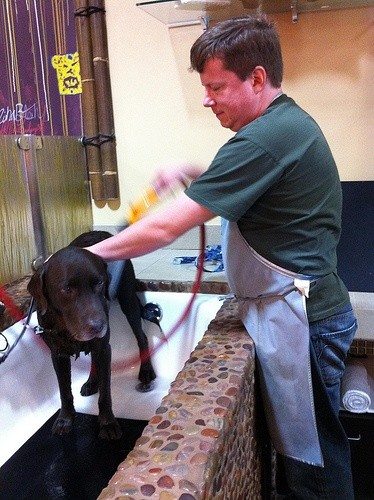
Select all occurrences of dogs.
[27,231,157,441]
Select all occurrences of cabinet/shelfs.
[136,0,374,32]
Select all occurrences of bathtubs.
[0,291,233,500]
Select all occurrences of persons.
[83,12,359,500]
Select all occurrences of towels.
[342,365,374,414]
